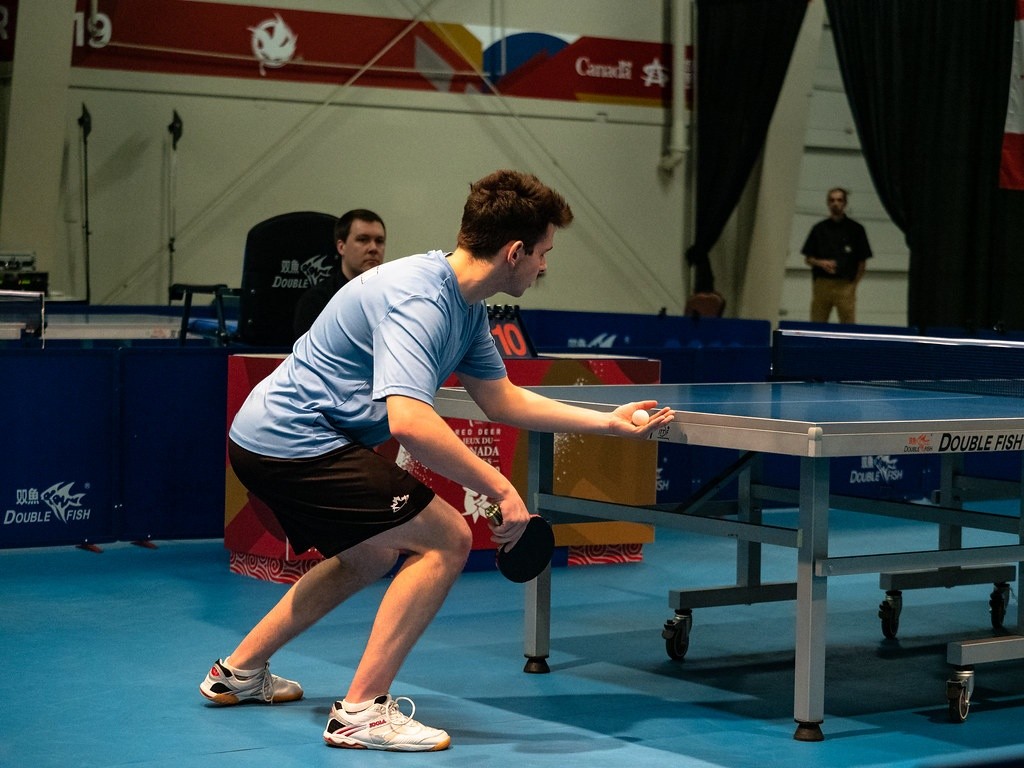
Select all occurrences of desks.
[430,378,1024,746]
[225,349,661,584]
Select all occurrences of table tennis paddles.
[485,503,556,584]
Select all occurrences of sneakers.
[198,658,304,707]
[323,694,451,753]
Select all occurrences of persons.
[294,209,387,341]
[198,170,676,752]
[800,188,873,324]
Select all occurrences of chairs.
[659,293,725,319]
[168,210,342,349]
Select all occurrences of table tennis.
[632,409,650,426]
